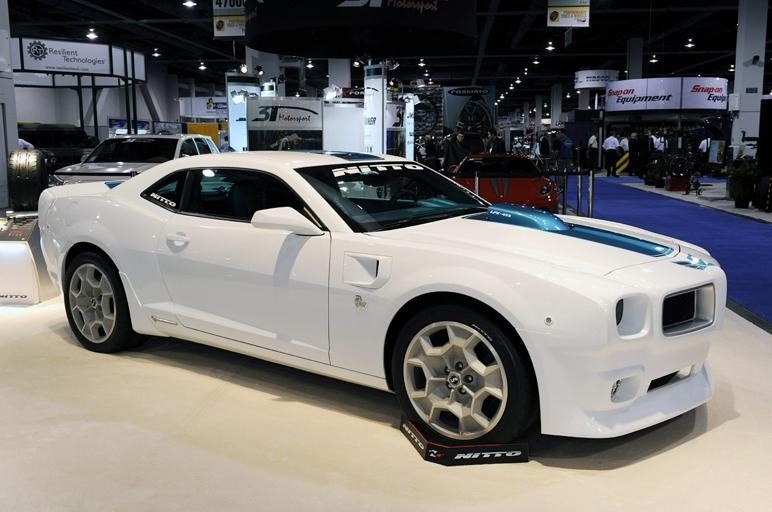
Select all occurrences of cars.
[50,133,221,187]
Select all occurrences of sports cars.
[37,150,729,449]
[445,151,560,213]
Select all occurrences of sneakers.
[606,172,620,177]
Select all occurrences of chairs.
[228,168,339,218]
[112,140,181,162]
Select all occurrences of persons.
[18,135,35,149]
[219,135,229,151]
[418,123,712,178]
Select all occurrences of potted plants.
[725,159,764,208]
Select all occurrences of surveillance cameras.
[752,48,761,64]
